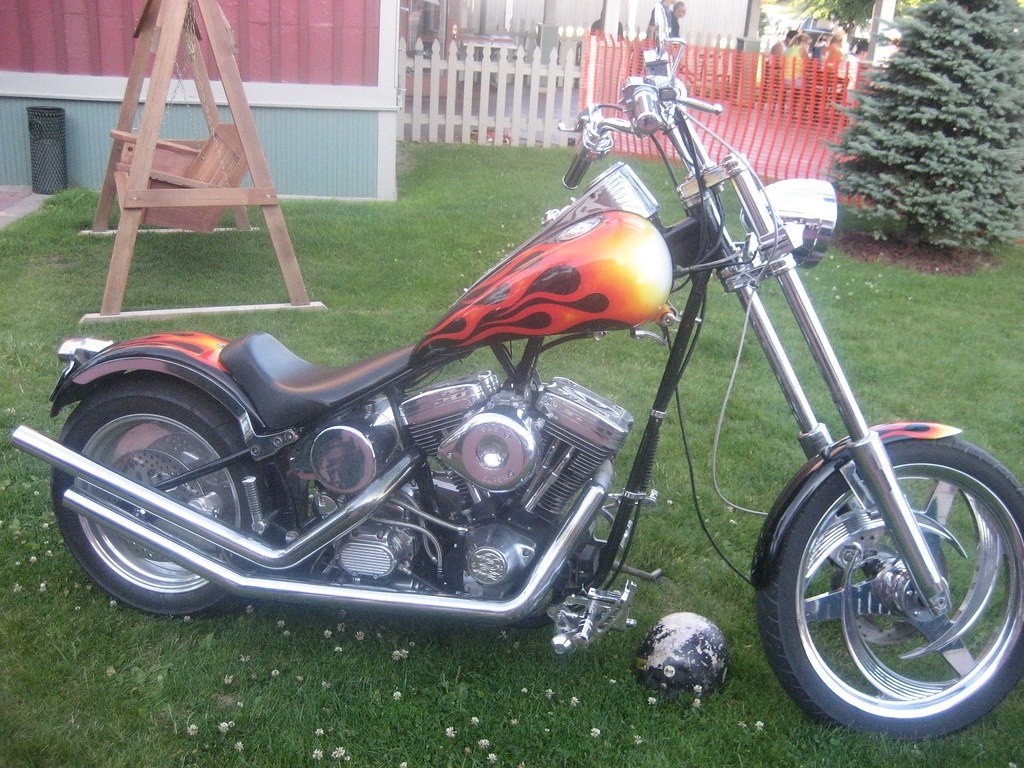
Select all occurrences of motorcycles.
[7,0,1024,741]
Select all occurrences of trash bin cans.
[25,105,69,196]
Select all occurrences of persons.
[590,10,624,39]
[646,0,686,44]
[766,21,902,132]
